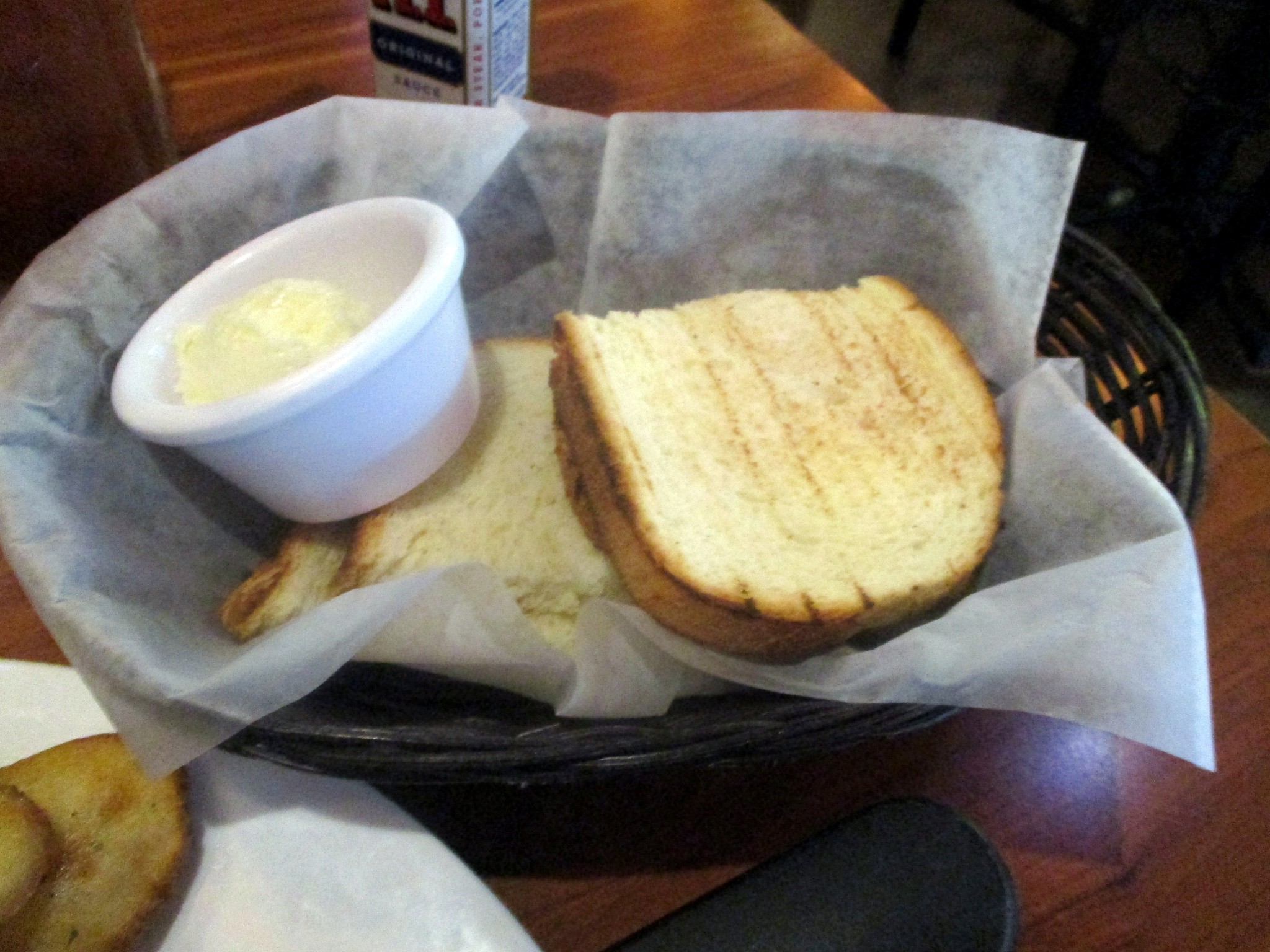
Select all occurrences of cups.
[110,196,480,523]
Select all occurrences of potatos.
[0,733,194,952]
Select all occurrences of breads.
[549,275,1005,652]
[221,337,619,645]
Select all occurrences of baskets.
[1,130,1212,792]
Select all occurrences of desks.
[1,2,1269,952]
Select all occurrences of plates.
[0,656,542,952]
